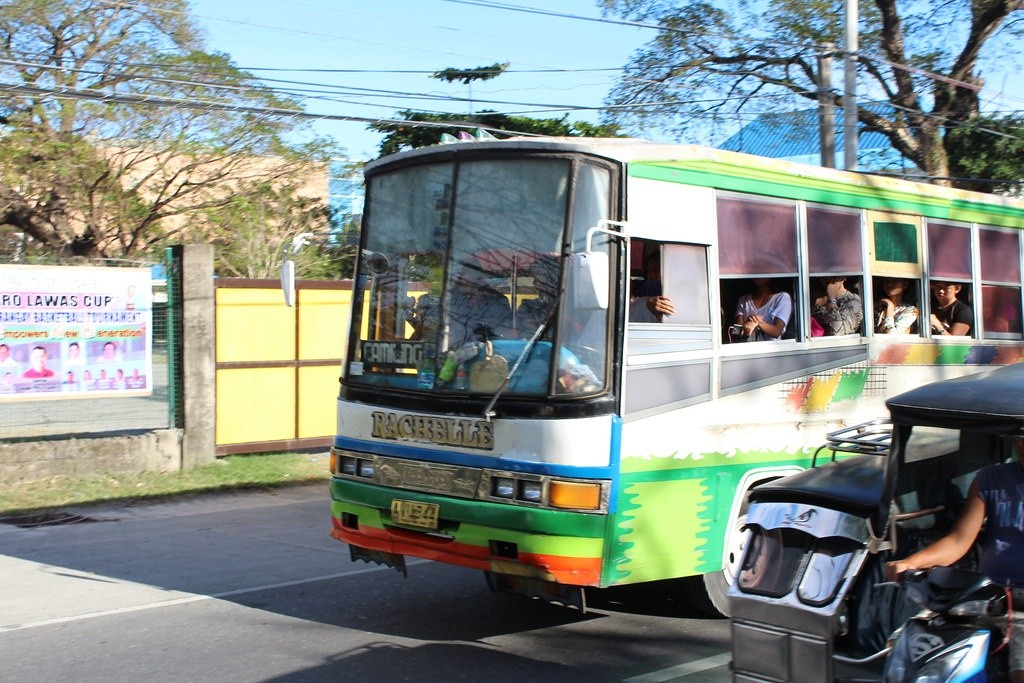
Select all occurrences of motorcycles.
[727,360,1023,683]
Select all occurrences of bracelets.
[938,329,944,335]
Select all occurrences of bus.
[278,128,1023,619]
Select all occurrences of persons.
[98,370,110,382]
[129,368,142,381]
[21,346,54,378]
[66,342,86,369]
[62,371,74,385]
[81,371,96,385]
[97,342,118,363]
[736,278,791,342]
[113,369,127,382]
[3,372,14,388]
[882,429,1024,612]
[874,277,919,335]
[0,344,16,368]
[403,251,674,352]
[930,281,973,336]
[812,276,863,336]
